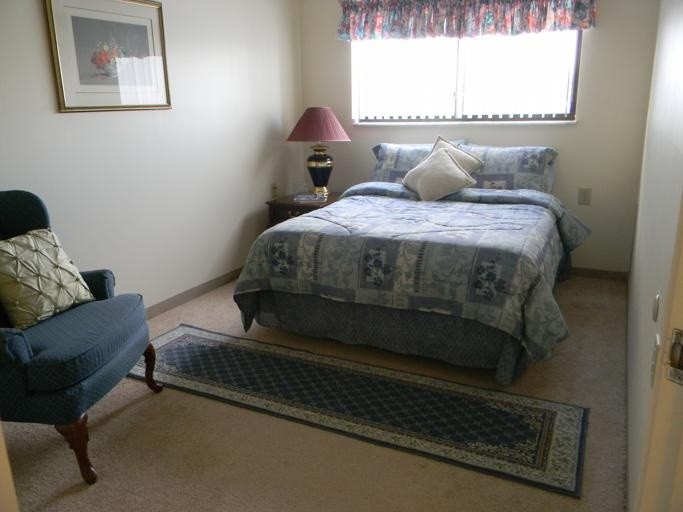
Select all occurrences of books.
[293,194,327,203]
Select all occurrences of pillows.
[368,132,557,205]
[1,224,95,330]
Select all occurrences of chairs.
[0,189,165,485]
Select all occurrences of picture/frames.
[42,0,172,113]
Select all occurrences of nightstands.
[265,188,353,226]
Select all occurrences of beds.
[254,142,567,386]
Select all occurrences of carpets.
[122,322,591,501]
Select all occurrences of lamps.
[284,105,352,199]
